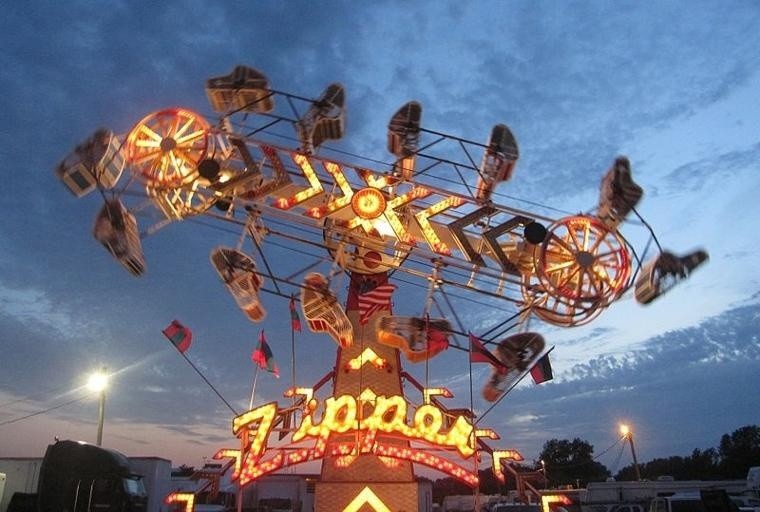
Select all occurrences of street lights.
[88,368,107,447]
[620,426,642,482]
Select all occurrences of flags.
[289,295,303,333]
[470,331,507,376]
[252,330,279,378]
[530,353,553,384]
[163,319,193,354]
[426,317,451,352]
[358,283,398,325]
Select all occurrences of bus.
[537,489,589,504]
[650,491,759,512]
[492,503,568,512]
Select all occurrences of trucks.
[37,440,148,511]
